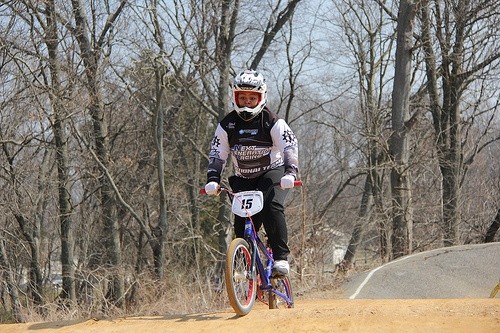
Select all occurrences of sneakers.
[235,269,247,280]
[274,260,290,274]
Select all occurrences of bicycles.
[199,182,303,316]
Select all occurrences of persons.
[205,68,299,277]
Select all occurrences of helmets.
[232,70,268,121]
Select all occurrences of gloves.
[205,181,218,195]
[281,175,295,190]
